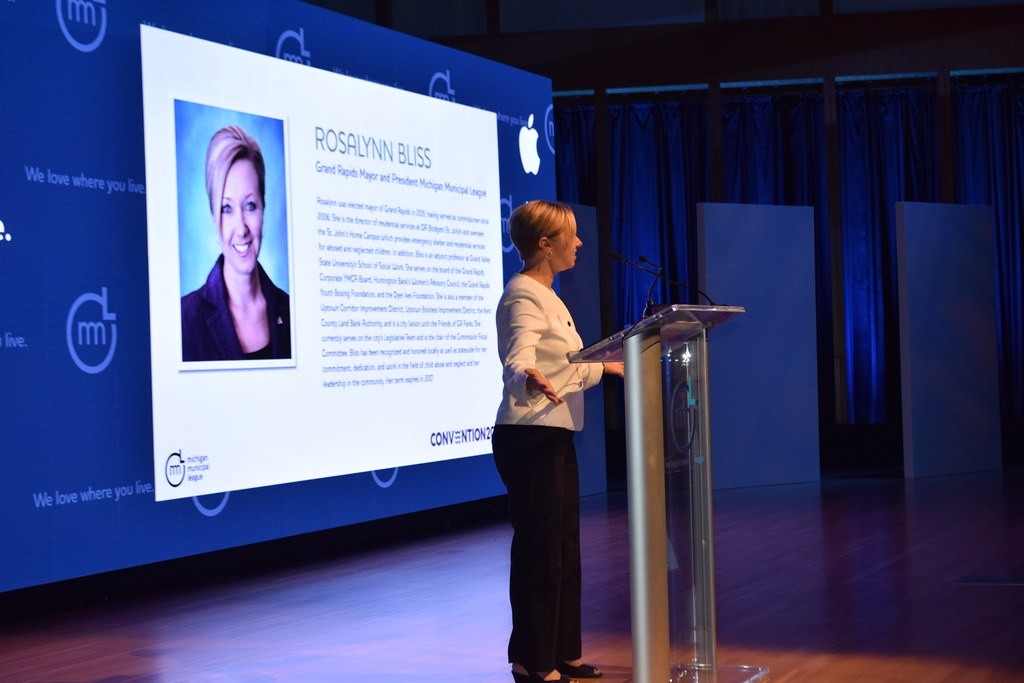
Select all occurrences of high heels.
[554,659,602,677]
[512,657,571,683]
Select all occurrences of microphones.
[608,252,717,322]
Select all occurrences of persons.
[494,202,623,683]
[181,125,291,361]
